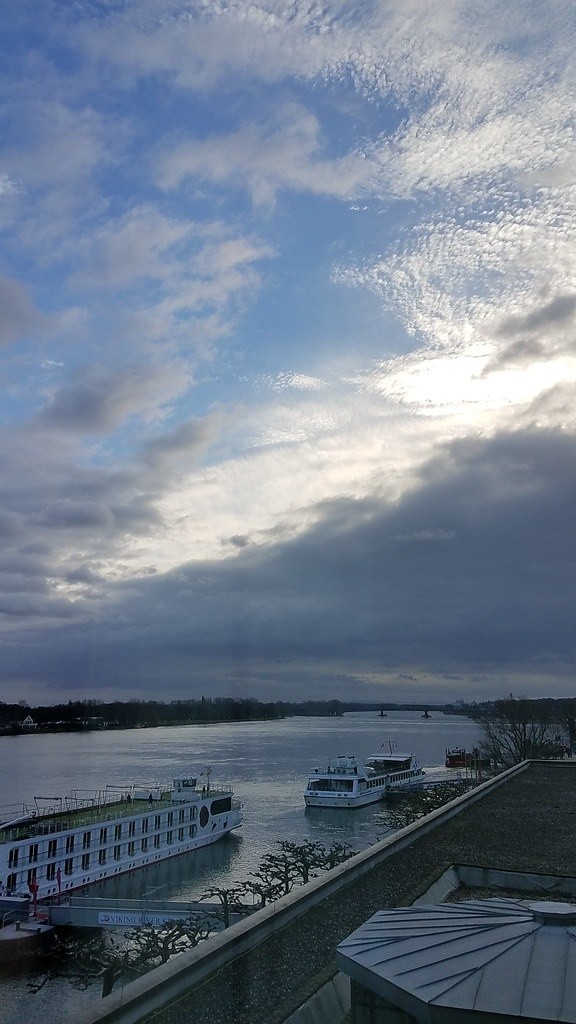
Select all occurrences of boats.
[0,775,245,908]
[303,753,428,808]
